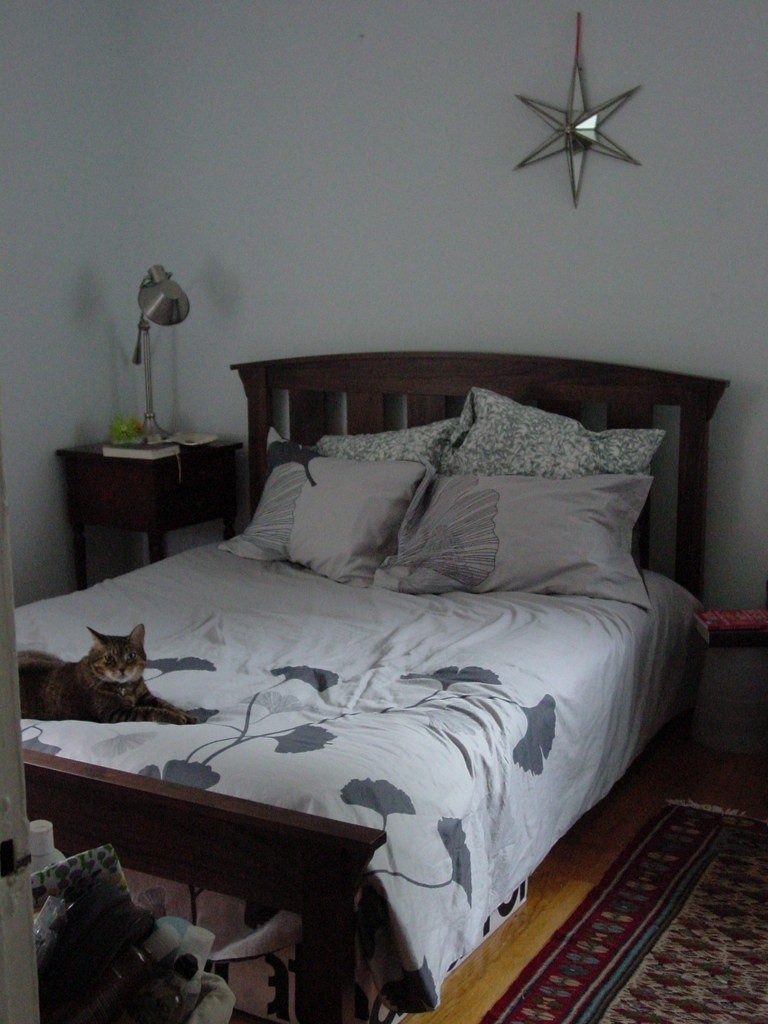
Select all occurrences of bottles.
[29,819,66,873]
[52,916,216,1024]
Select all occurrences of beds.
[14,350,731,1024]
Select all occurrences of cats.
[17,624,199,725]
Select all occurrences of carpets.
[479,798,768,1024]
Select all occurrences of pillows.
[218,427,435,589]
[371,467,655,609]
[434,386,666,480]
[310,417,459,466]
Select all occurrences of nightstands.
[55,441,243,591]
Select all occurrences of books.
[693,607,768,647]
[102,442,180,460]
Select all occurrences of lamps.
[131,263,189,444]
